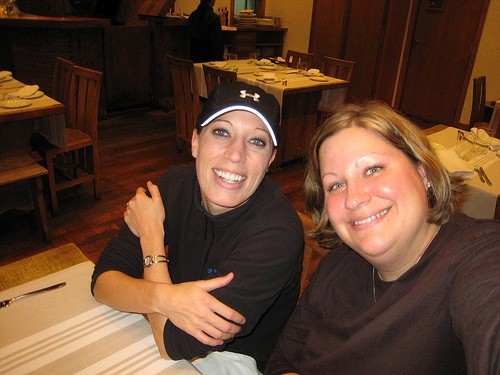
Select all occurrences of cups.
[249,52,257,64]
[276,64,287,80]
[229,54,237,67]
[297,62,307,76]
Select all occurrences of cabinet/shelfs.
[8,25,286,111]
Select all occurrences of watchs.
[143,255,169,267]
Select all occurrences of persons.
[90,81,304,373]
[188,0,224,63]
[263,99,500,375]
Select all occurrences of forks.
[0,281,66,308]
[474,168,485,183]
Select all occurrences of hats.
[199,81,281,146]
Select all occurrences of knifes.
[479,167,492,186]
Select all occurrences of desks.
[0,9,110,28]
[0,242,203,375]
[422,124,500,220]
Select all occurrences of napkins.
[263,73,276,80]
[15,85,39,97]
[256,59,271,65]
[470,128,500,151]
[213,61,226,68]
[307,69,320,76]
[0,71,12,80]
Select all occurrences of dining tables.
[0,79,65,149]
[192,58,349,171]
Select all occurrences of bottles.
[216,6,228,26]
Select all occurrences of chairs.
[285,50,314,70]
[298,207,332,295]
[165,53,204,160]
[51,57,76,176]
[316,55,356,127]
[28,65,103,227]
[202,64,237,97]
[469,76,494,138]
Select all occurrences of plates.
[0,76,44,108]
[205,59,329,84]
[235,9,258,26]
